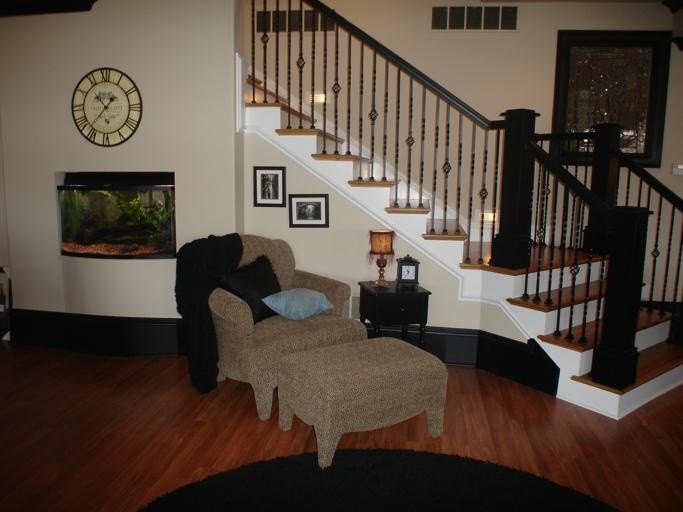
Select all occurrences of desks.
[357,280,432,345]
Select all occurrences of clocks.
[70,67,144,148]
[395,253,420,287]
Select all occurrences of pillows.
[261,288,333,321]
[220,254,282,325]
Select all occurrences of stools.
[275,335,448,471]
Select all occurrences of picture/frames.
[252,165,287,208]
[549,30,673,169]
[288,193,330,229]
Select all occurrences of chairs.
[172,232,369,422]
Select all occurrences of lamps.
[369,229,395,288]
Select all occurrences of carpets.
[140,446,615,512]
